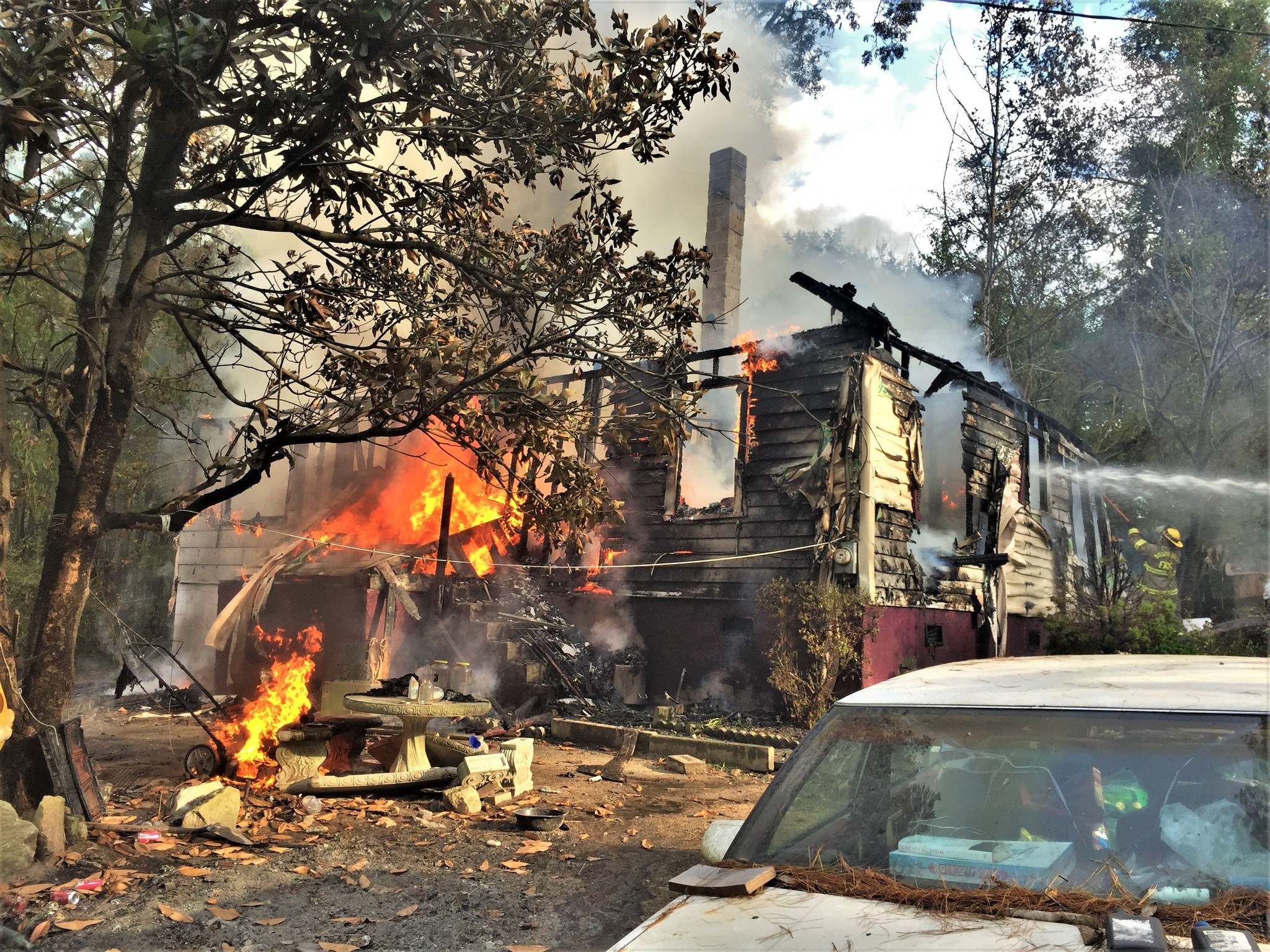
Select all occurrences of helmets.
[1163,528,1183,548]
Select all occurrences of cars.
[604,651,1270,952]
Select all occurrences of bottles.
[450,662,472,695]
[1104,907,1169,952]
[418,678,434,705]
[429,660,450,692]
[1148,886,1210,907]
[1190,921,1262,952]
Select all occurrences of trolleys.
[126,641,246,782]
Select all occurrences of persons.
[1126,520,1189,634]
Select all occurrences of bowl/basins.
[512,808,569,832]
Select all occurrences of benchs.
[277,712,382,788]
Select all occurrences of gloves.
[1127,522,1135,529]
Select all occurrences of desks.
[346,693,490,771]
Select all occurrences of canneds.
[135,831,162,843]
[50,891,79,904]
[74,880,104,890]
